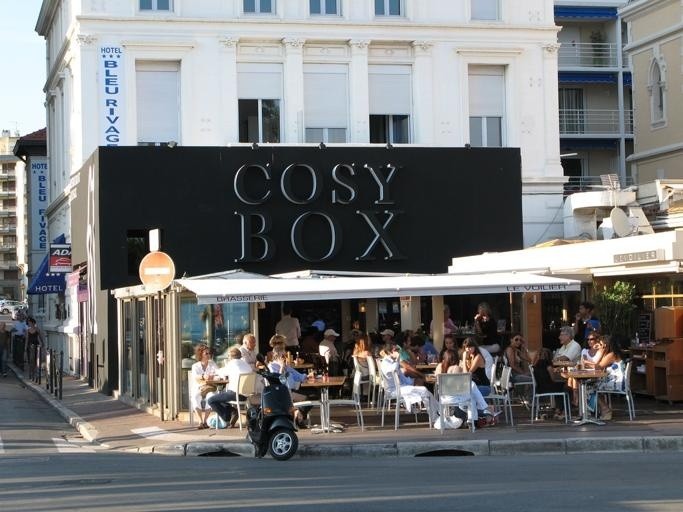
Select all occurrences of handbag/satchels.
[206,415,226,429]
[434,414,464,430]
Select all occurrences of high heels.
[295,416,307,429]
[483,409,504,420]
[466,419,476,433]
[599,410,612,421]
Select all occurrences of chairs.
[215,363,364,434]
[570,361,635,424]
[529,365,571,426]
[353,352,533,433]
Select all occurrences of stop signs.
[139,251,175,292]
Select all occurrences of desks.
[449,334,476,337]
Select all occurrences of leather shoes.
[198,423,209,429]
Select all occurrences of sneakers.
[223,409,239,428]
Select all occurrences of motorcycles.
[245,353,299,461]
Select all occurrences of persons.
[0,322,9,377]
[11,314,28,366]
[25,319,44,375]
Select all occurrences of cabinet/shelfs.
[627,341,683,407]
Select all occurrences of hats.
[380,329,395,336]
[324,328,340,337]
[312,320,326,331]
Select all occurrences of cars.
[0,298,28,321]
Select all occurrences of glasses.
[515,337,522,342]
